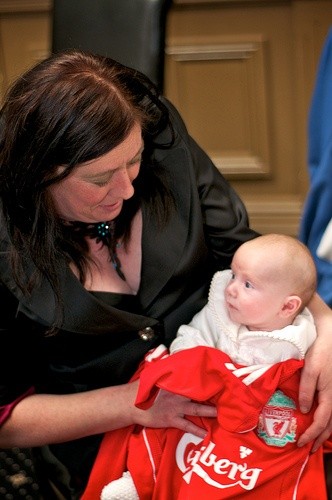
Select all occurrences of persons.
[100,233,317,500]
[1,51,332,500]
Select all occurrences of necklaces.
[70,220,126,280]
